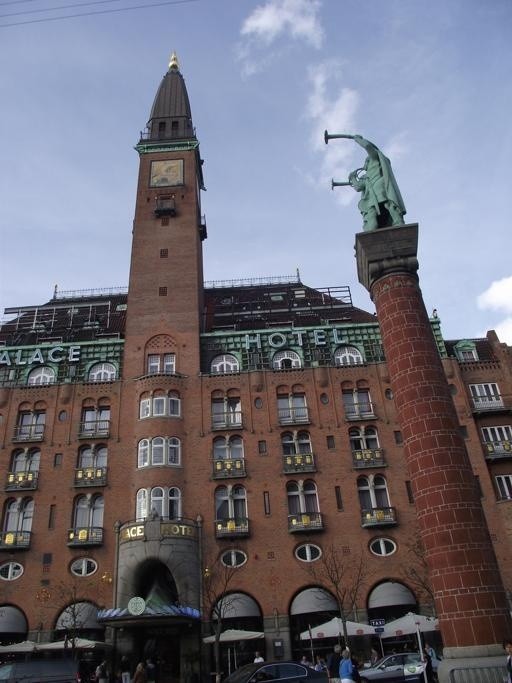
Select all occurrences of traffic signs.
[374,628,384,633]
[369,618,386,626]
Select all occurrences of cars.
[220,659,331,683]
[357,650,441,682]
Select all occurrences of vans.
[0,656,81,683]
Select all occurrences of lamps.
[101,571,112,584]
[204,568,211,577]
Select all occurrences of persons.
[249,639,442,682]
[94,653,157,683]
[502,637,511,682]
[345,135,404,229]
